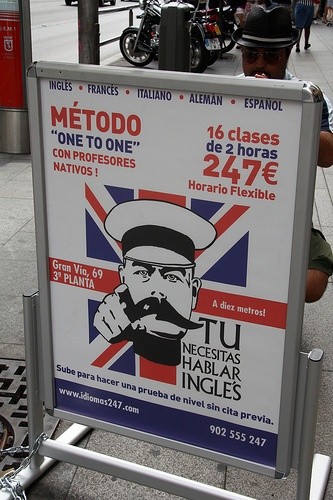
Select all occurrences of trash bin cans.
[156,2,196,71]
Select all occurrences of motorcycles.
[118,0,240,74]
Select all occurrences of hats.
[230,5,300,50]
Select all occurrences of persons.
[231,4,333,303]
[231,0,333,48]
[294,0,314,52]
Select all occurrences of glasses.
[241,51,288,65]
